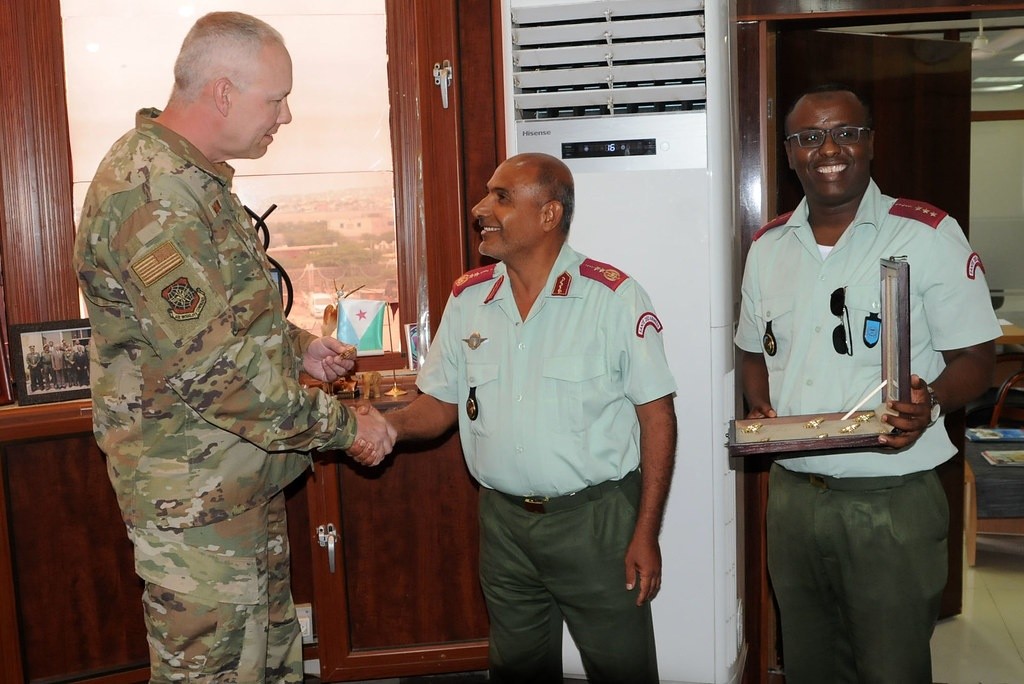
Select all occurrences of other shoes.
[32,383,78,391]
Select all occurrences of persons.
[733,84,1004,684]
[72,11,398,684]
[345,153,678,684]
[26,339,91,392]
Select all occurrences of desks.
[964,428,1024,566]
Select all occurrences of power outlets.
[293,603,314,644]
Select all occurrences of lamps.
[972,19,990,50]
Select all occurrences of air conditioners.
[499,0,747,684]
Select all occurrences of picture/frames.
[8,318,93,408]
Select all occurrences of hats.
[44,344,50,348]
[29,345,36,348]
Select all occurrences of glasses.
[830,286,855,357]
[787,126,871,148]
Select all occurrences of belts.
[492,471,633,514]
[773,461,927,489]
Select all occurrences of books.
[965,428,1024,442]
[981,451,1024,466]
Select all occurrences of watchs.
[927,385,940,427]
